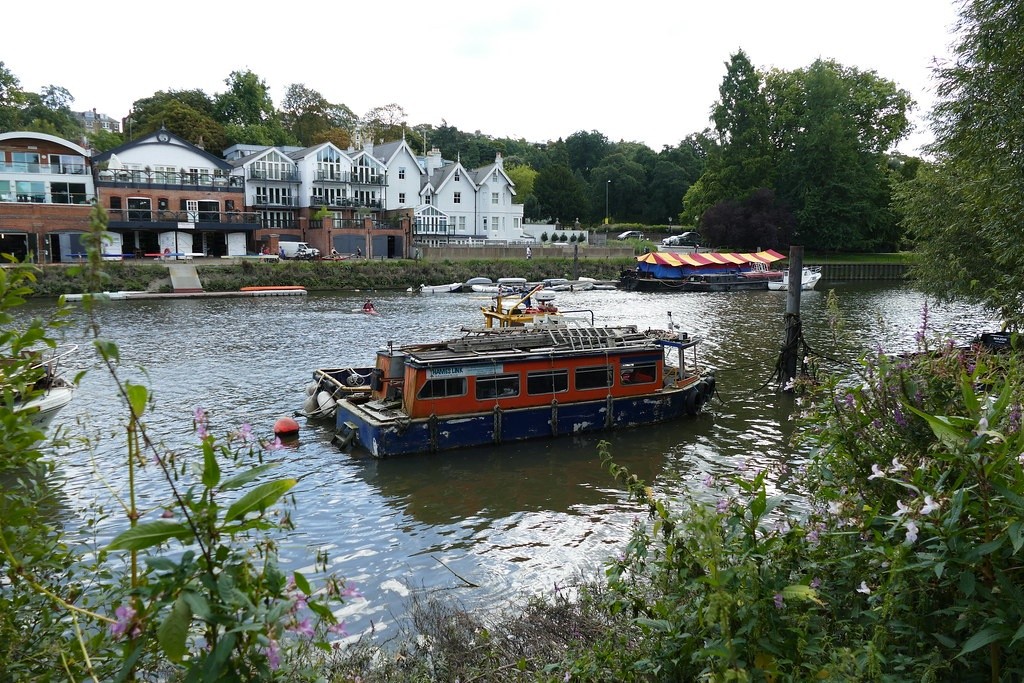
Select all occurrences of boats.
[621,249,783,291]
[418,282,463,293]
[311,283,714,460]
[767,267,822,291]
[471,282,516,293]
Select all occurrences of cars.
[617,230,645,242]
[663,232,703,247]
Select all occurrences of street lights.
[669,216,672,237]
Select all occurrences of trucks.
[278,241,320,260]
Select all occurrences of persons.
[644,246,649,254]
[527,246,532,259]
[280,246,285,260]
[331,247,340,258]
[694,243,698,252]
[357,246,362,258]
[258,244,270,263]
[362,299,374,311]
[297,249,302,260]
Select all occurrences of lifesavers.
[686,390,703,413]
[164,248,171,258]
[705,376,717,403]
[698,381,708,407]
